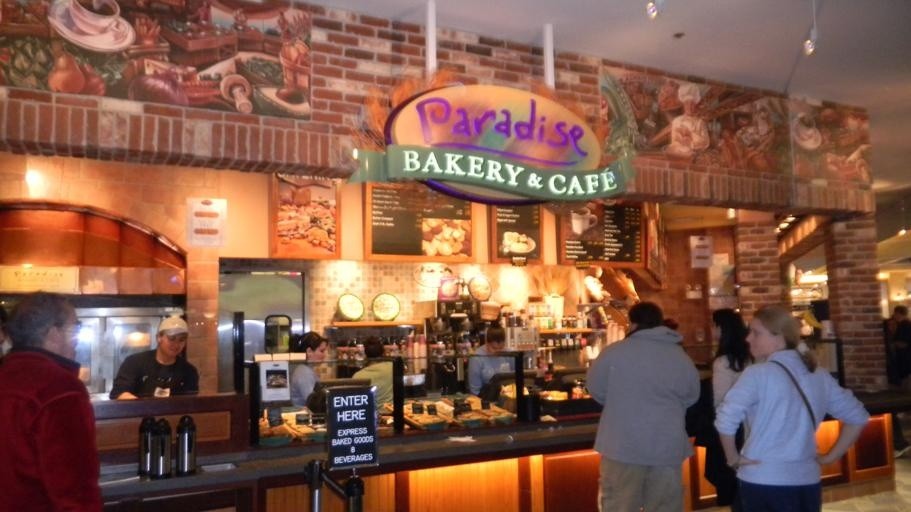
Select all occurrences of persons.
[587,301,701,512]
[465,320,516,397]
[107,315,199,400]
[711,307,758,512]
[350,336,407,415]
[884,317,899,335]
[1,290,106,512]
[886,306,911,395]
[609,67,872,189]
[287,330,330,424]
[708,304,872,512]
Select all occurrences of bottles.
[338,325,431,362]
[176,415,197,477]
[498,303,605,369]
[138,416,156,476]
[151,418,172,480]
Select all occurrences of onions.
[127,68,188,108]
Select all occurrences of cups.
[571,207,599,236]
[797,112,817,140]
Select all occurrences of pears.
[48,45,85,93]
[79,57,106,97]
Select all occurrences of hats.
[159,317,188,337]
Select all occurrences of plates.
[503,235,537,254]
[792,125,822,151]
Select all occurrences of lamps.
[806,0,818,54]
[647,0,666,18]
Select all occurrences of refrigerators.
[217,269,307,393]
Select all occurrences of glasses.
[54,320,82,329]
